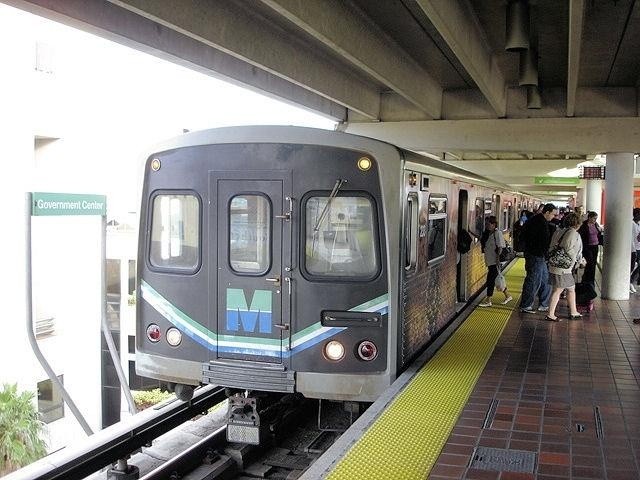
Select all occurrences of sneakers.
[633,316,640,325]
[479,292,595,322]
[629,282,636,293]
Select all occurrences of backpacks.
[458,228,473,254]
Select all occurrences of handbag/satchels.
[498,246,515,263]
[575,283,597,305]
[549,247,573,269]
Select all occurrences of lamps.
[505,0,544,110]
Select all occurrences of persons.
[477,217,513,307]
[428,199,445,259]
[512,202,640,320]
[455,227,461,265]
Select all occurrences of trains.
[132,123,543,447]
